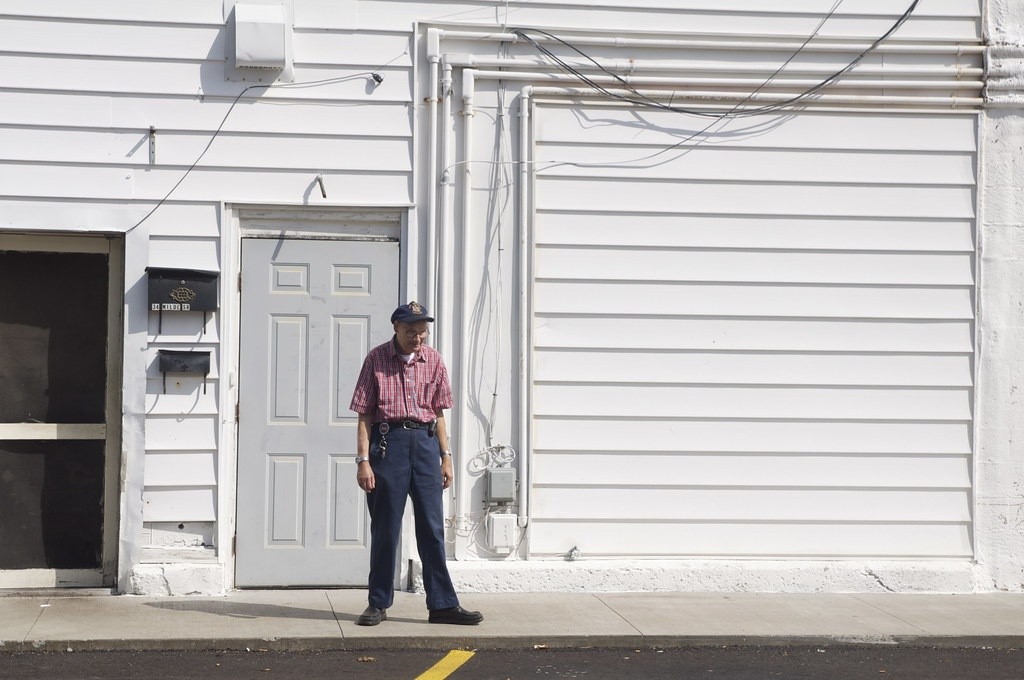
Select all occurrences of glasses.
[401,327,430,338]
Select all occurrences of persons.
[349,301,484,624]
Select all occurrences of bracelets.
[440,450,451,456]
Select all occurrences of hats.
[392,301,433,325]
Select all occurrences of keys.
[380,446,385,458]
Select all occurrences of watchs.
[354,456,368,464]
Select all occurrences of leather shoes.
[430,607,485,625]
[358,605,386,625]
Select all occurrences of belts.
[373,421,435,431]
